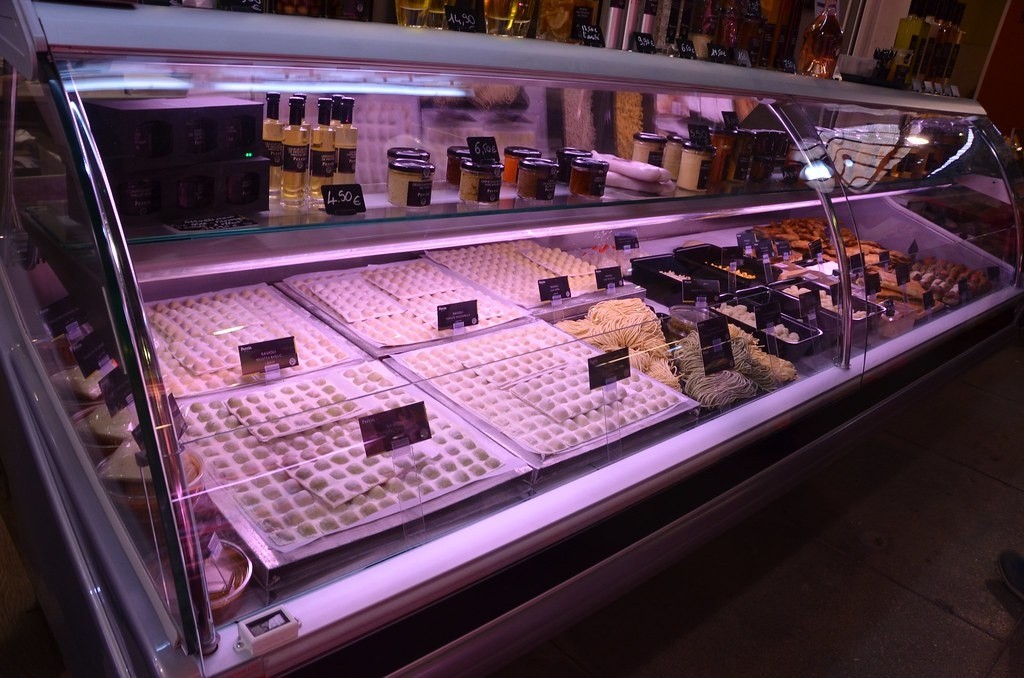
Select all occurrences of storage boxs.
[67,90,271,229]
[630,242,886,363]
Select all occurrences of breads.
[751,214,996,305]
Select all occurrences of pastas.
[552,298,797,411]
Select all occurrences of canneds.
[387,145,609,207]
[630,128,785,192]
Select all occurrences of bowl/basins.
[73,404,143,457]
[97,448,205,523]
[143,538,253,624]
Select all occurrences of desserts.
[67,358,156,495]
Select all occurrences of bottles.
[333,97,357,189]
[292,94,309,199]
[262,93,283,196]
[281,95,308,206]
[307,97,335,206]
[330,95,345,129]
[796,5,844,80]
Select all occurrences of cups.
[484,0,536,39]
[394,0,456,31]
